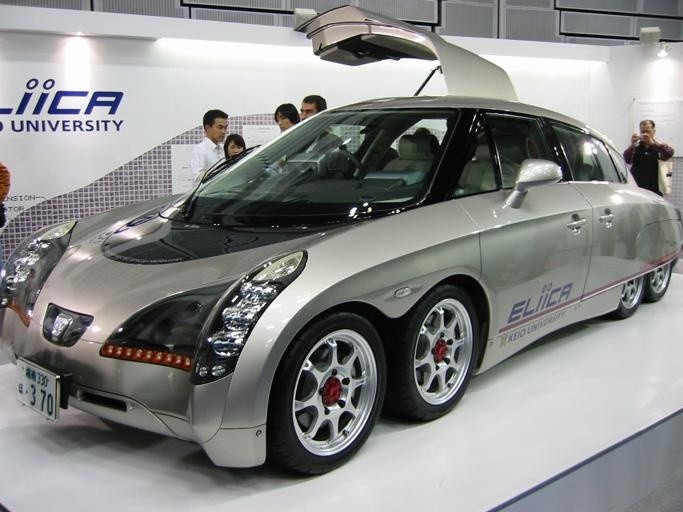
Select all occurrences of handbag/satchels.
[657,159,673,195]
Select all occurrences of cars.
[0,5,682,475]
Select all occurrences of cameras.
[637,135,645,141]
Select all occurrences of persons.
[223,134,247,161]
[190,110,229,187]
[623,119,675,196]
[23,267,37,308]
[288,95,347,157]
[275,104,301,131]
